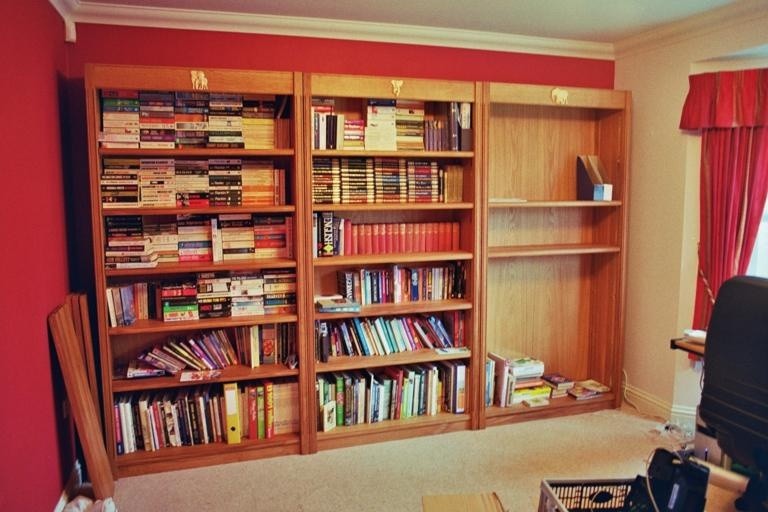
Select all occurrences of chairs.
[698,275,767,472]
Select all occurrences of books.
[316,361,466,432]
[313,211,460,258]
[313,158,464,203]
[313,261,468,313]
[98,89,292,149]
[315,310,470,363]
[113,323,296,382]
[101,159,285,206]
[113,381,299,455]
[104,213,292,268]
[106,270,300,328]
[312,98,471,151]
[485,352,611,408]
[489,197,527,202]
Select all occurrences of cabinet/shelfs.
[84,62,310,481]
[303,72,482,455]
[479,82,633,431]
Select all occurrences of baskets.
[538,477,635,512]
